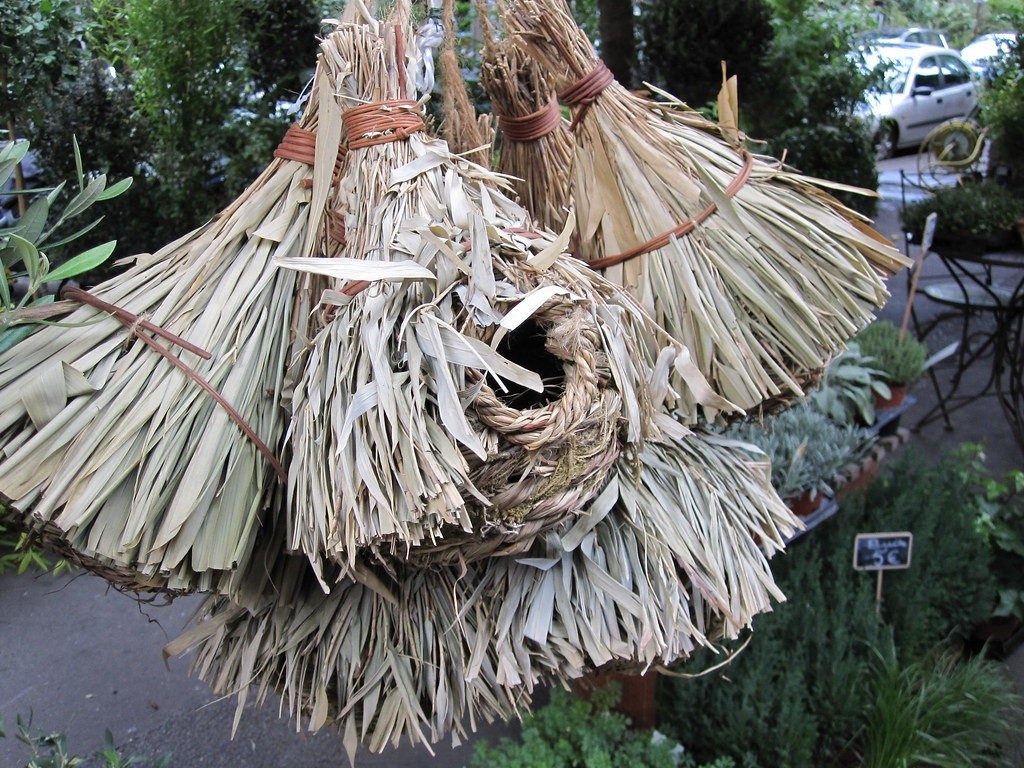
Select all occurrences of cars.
[808,9,1023,163]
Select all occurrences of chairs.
[896,168,1024,457]
